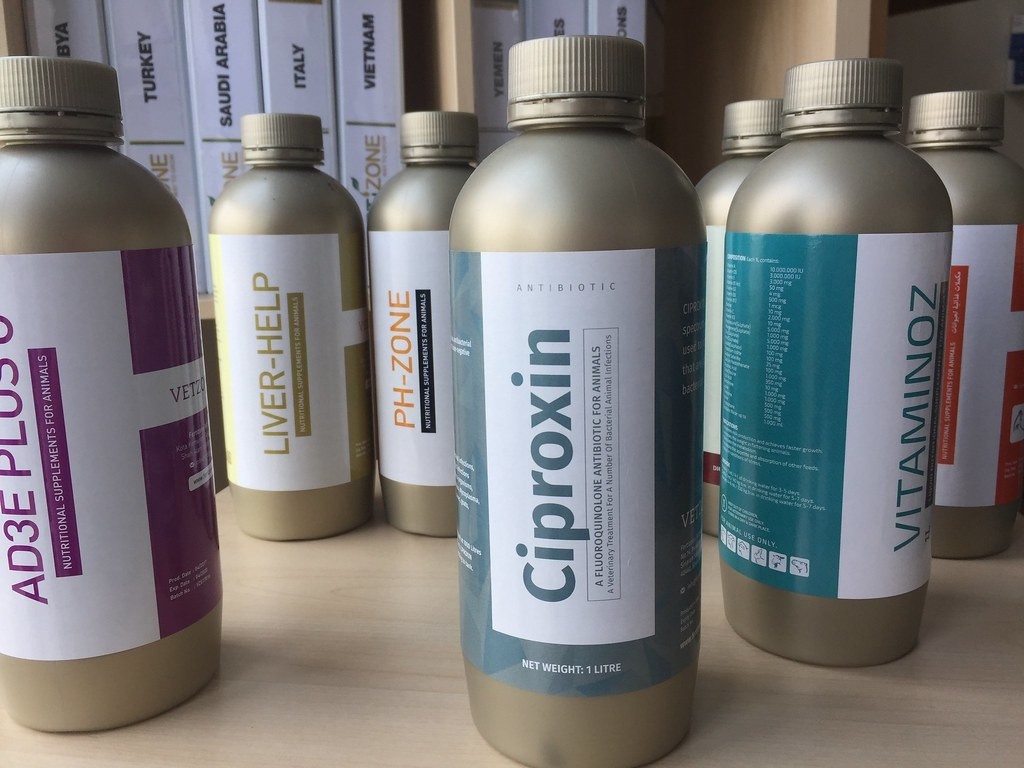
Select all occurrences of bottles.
[367,112,482,538]
[717,59,931,667]
[694,98,784,540]
[450,36,704,768]
[906,89,1024,562]
[0,55,223,732]
[210,114,371,541]
